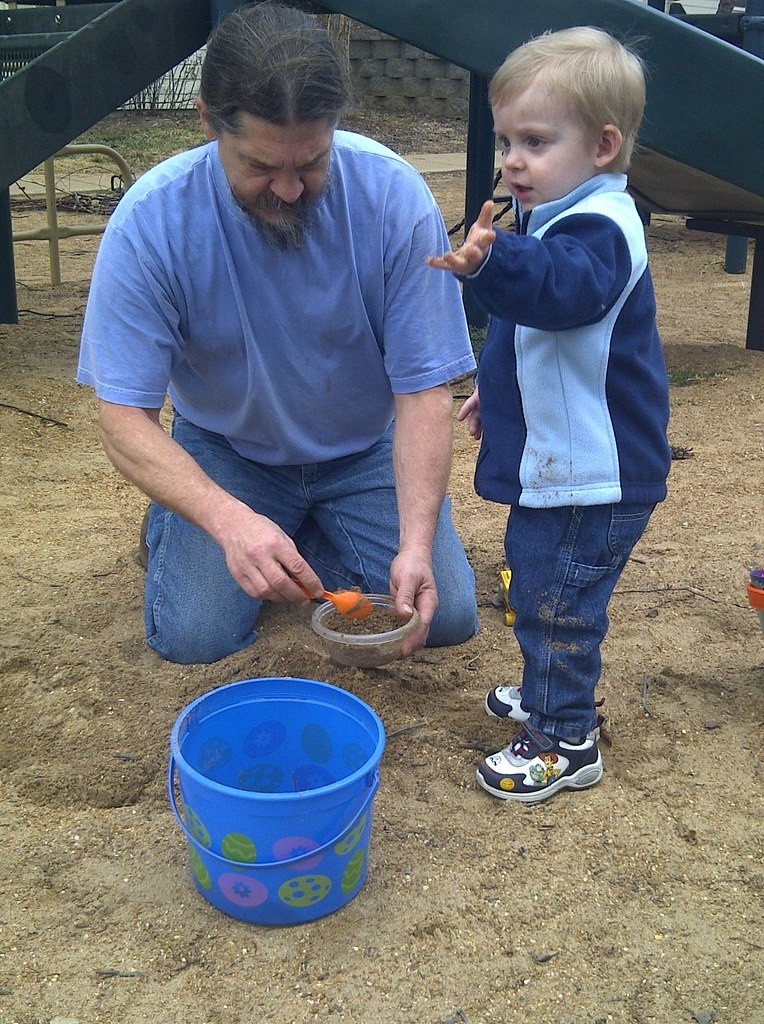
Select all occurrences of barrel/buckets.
[168,678,385,928]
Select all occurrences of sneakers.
[487,686,539,723]
[477,722,603,803]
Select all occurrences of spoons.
[293,578,373,620]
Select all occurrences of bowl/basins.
[310,595,419,666]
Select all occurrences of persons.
[74,2,480,664]
[424,25,672,802]
